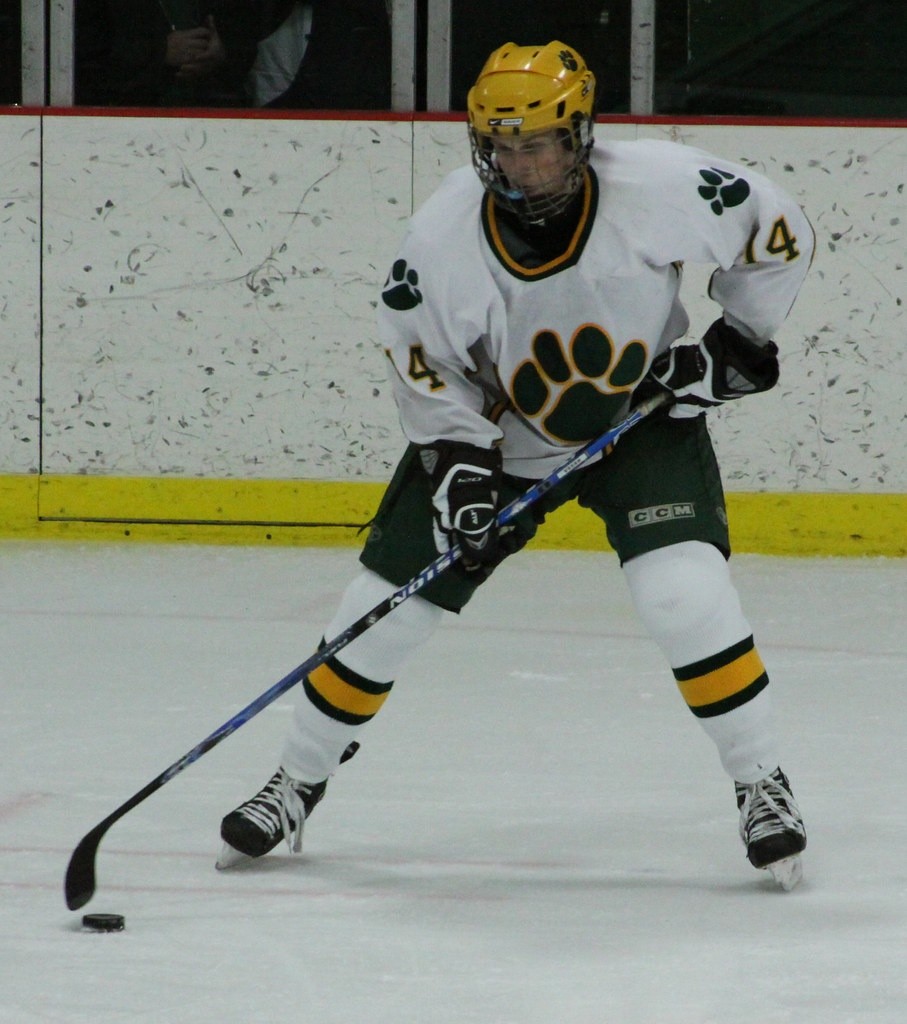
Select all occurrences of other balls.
[81,912,126,934]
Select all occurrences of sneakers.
[734,766,808,890]
[215,741,360,868]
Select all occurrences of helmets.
[466,41,595,227]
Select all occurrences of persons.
[215,40,816,892]
[82,0,232,106]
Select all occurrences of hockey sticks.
[59,392,673,915]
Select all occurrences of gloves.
[413,440,527,577]
[645,317,779,422]
